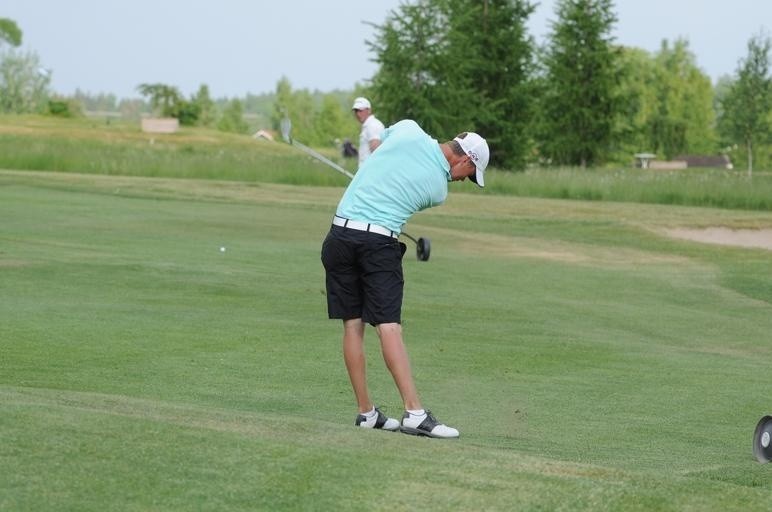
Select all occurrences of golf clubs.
[281,119,354,180]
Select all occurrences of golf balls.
[220,247,225,252]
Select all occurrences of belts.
[331,215,399,240]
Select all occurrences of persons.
[320,116,491,440]
[351,96,385,170]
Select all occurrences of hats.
[453,131,490,188]
[351,97,371,110]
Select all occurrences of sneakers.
[400,408,460,439]
[355,406,401,432]
